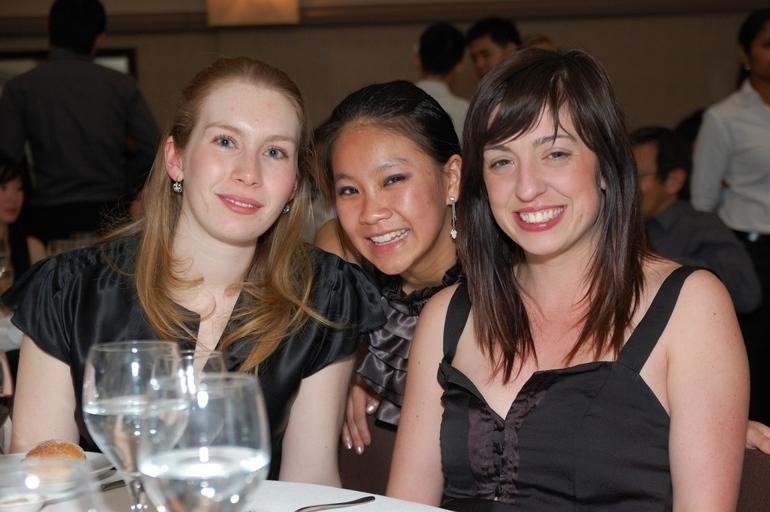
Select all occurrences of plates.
[0,445,114,491]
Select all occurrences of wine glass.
[132,373,272,512]
[150,350,228,450]
[81,341,190,512]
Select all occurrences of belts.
[731,229,770,243]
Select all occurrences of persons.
[413,23,472,152]
[1,162,48,292]
[691,11,770,311]
[463,15,522,74]
[10,57,362,488]
[626,126,763,314]
[1,3,162,274]
[385,45,752,512]
[312,79,478,496]
